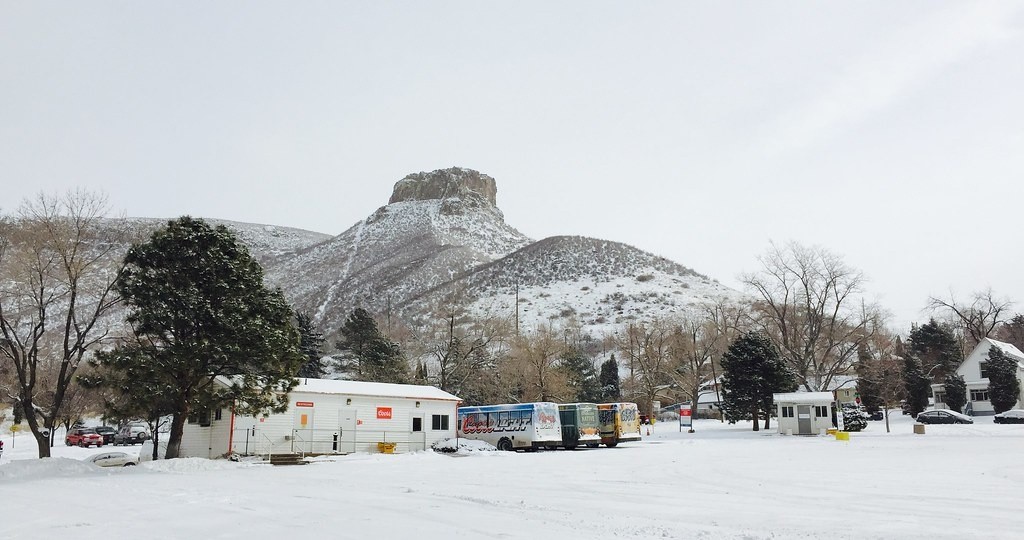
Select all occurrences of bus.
[457,402,563,452]
[557,401,602,450]
[597,400,641,448]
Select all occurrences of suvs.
[113,424,148,446]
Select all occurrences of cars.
[94,425,118,443]
[993,410,1024,424]
[916,409,974,426]
[65,428,104,448]
